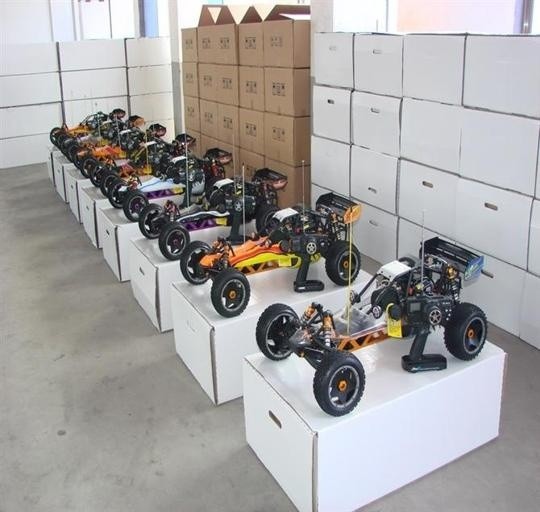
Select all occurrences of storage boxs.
[0,5,539,512]
